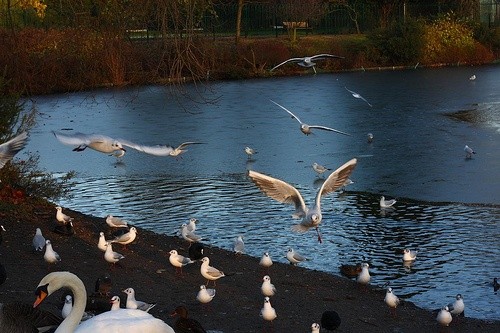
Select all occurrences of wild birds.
[166,250,198,271]
[402,247,419,261]
[344,86,372,108]
[51,130,172,157]
[0,131,500,333]
[312,162,332,177]
[380,196,396,207]
[469,75,476,80]
[247,157,357,245]
[464,145,476,159]
[367,132,373,142]
[108,226,138,245]
[259,251,274,270]
[270,53,343,71]
[199,256,226,287]
[244,145,259,159]
[106,214,129,228]
[271,100,351,137]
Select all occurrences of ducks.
[339,262,363,278]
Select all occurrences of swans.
[32,270,175,333]
